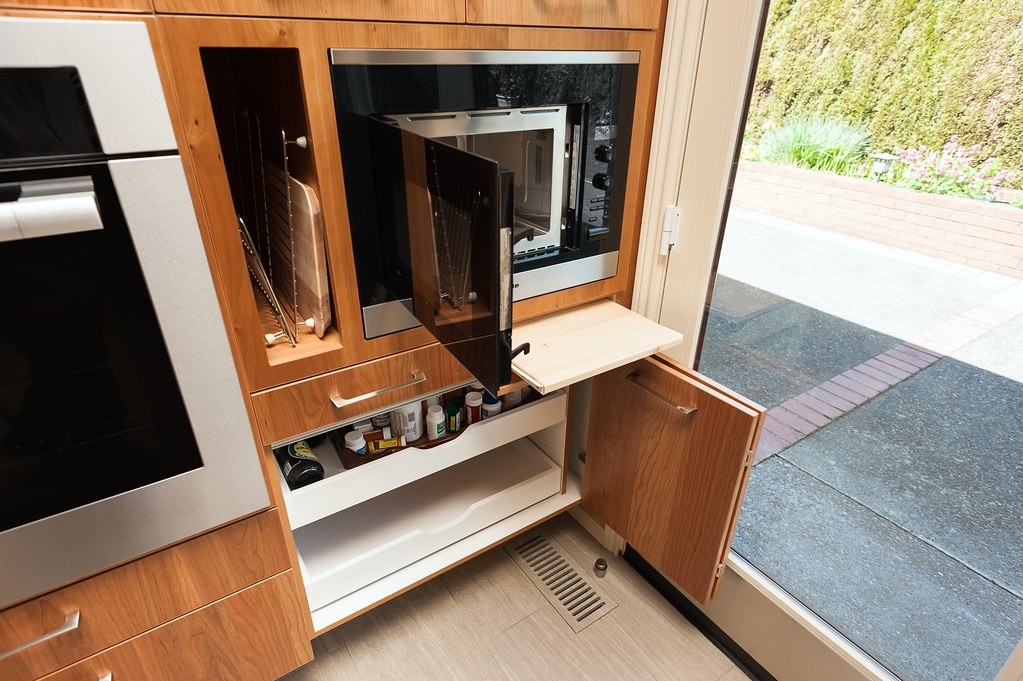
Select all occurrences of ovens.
[0,17,272,610]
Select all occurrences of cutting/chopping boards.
[266,159,332,338]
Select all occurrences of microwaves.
[326,49,641,399]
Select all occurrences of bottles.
[452,396,465,425]
[465,392,482,425]
[372,413,390,430]
[363,426,392,441]
[344,431,367,455]
[390,401,423,442]
[367,436,407,455]
[482,392,502,420]
[273,440,325,491]
[425,405,447,441]
[443,404,461,433]
[466,382,485,396]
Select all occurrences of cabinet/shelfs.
[0,4,767,681]
